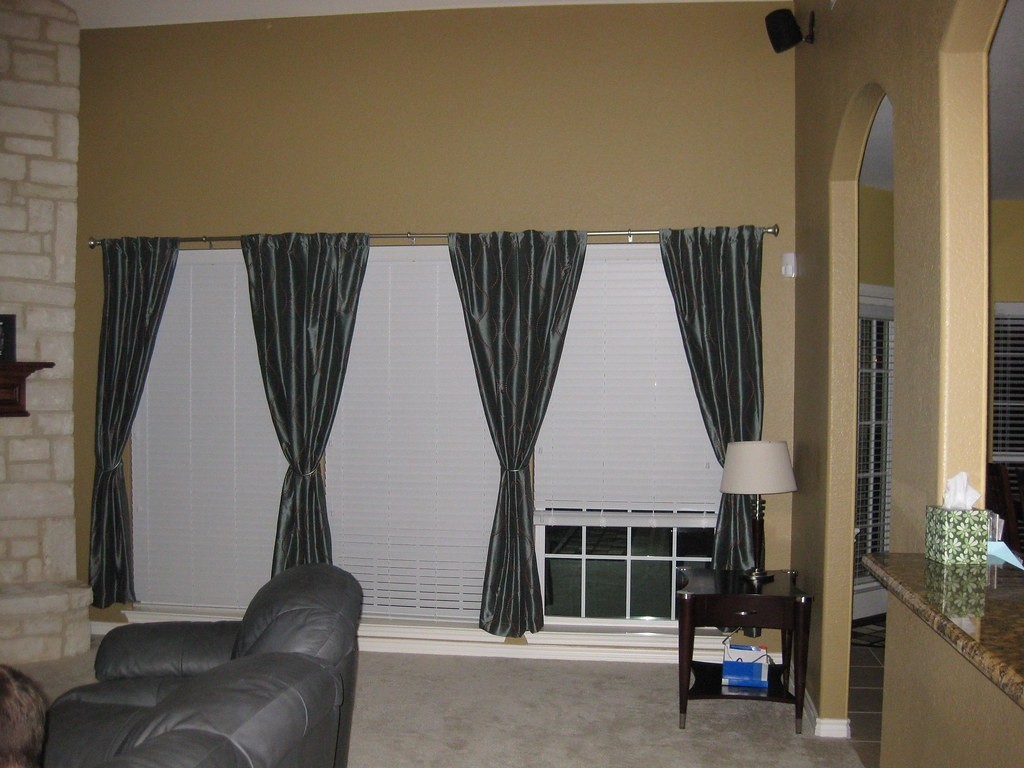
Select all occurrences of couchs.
[44,564,364,768]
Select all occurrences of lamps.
[721,442,796,582]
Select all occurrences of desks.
[677,568,810,734]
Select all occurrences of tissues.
[923,471,990,567]
